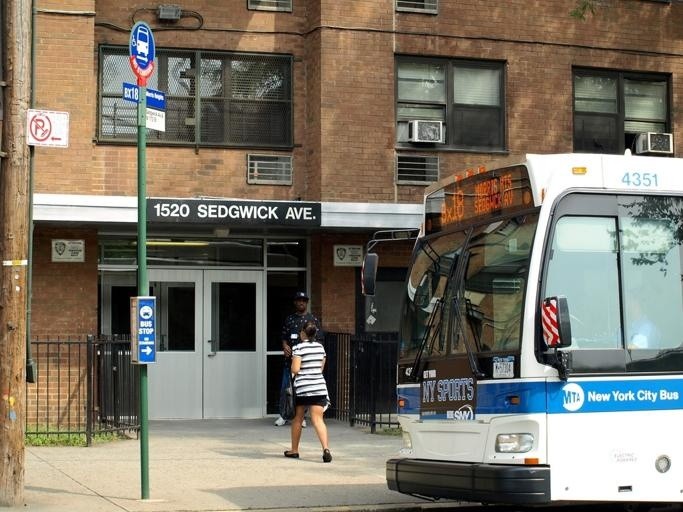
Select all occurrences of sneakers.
[302,420,307,428]
[323,449,332,462]
[274,416,286,426]
[284,451,299,458]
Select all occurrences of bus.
[359,146,683,512]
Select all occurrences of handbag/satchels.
[281,387,296,419]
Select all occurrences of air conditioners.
[407,120,443,144]
[635,131,674,155]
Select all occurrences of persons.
[273,293,325,427]
[611,290,662,351]
[283,320,333,462]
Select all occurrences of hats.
[295,292,309,300]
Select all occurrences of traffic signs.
[121,80,167,112]
[135,296,157,364]
[144,107,167,134]
